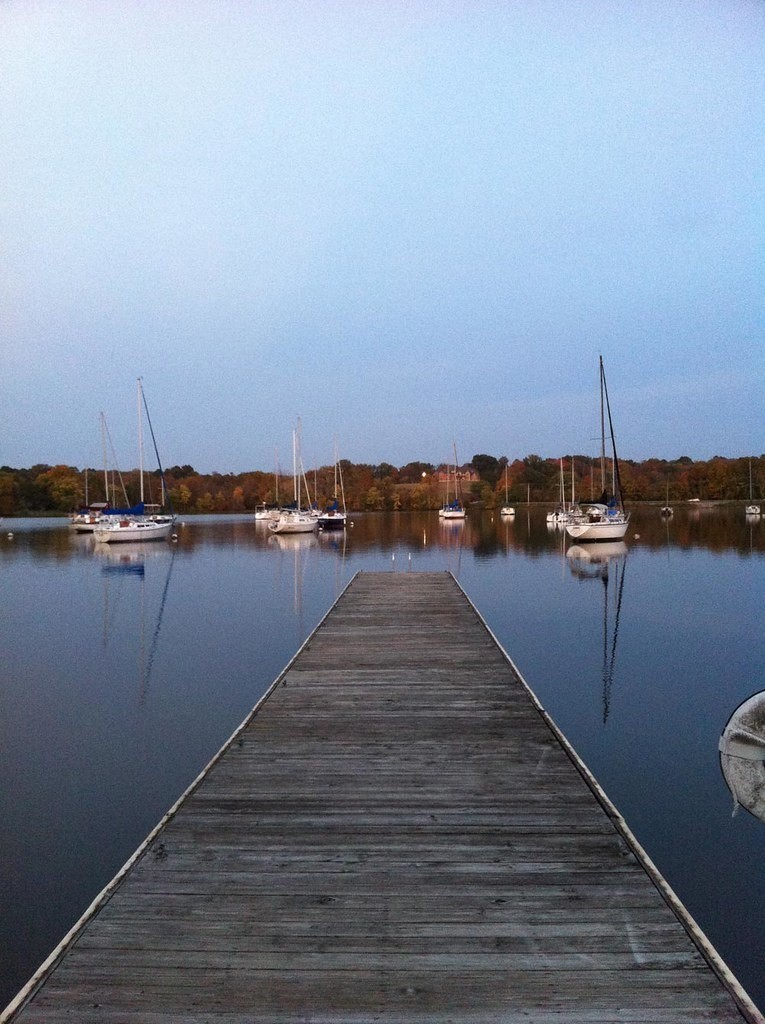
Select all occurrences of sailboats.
[71,410,131,533]
[309,434,349,529]
[96,537,171,709]
[254,444,284,521]
[438,441,466,520]
[545,355,630,541]
[500,465,516,516]
[93,378,177,545]
[566,540,632,729]
[268,415,320,535]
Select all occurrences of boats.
[742,504,762,515]
[661,506,673,517]
